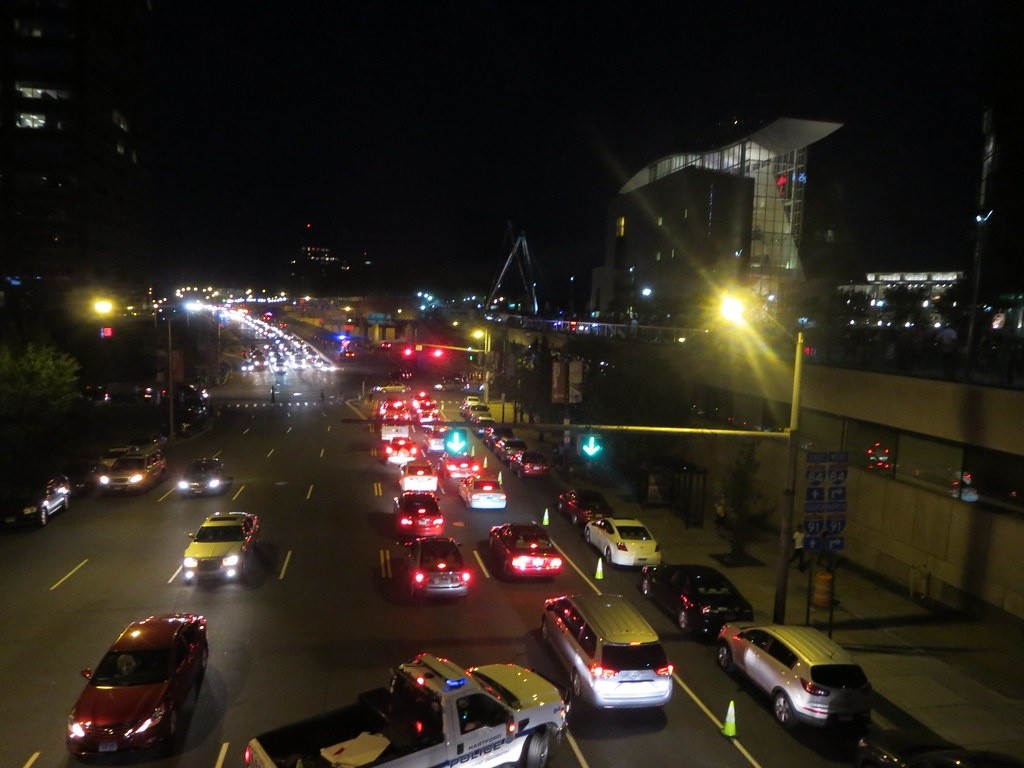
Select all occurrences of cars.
[857,726,1024,767]
[639,562,754,640]
[100,444,140,470]
[556,488,664,571]
[457,393,550,476]
[336,350,357,362]
[369,367,508,600]
[242,332,329,375]
[184,511,260,580]
[0,470,73,529]
[490,521,565,581]
[179,458,227,497]
[67,613,209,764]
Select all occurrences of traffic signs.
[806,451,850,551]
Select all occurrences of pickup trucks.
[243,653,570,768]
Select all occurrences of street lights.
[167,299,204,444]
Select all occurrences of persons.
[118,654,135,676]
[631,316,638,339]
[936,325,956,357]
[714,492,726,529]
[319,388,325,404]
[459,708,481,732]
[367,388,375,404]
[787,524,805,573]
[271,385,276,400]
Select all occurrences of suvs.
[102,449,168,498]
[716,619,877,731]
[541,593,674,711]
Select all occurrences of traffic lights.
[402,347,413,358]
[575,433,607,460]
[443,427,468,457]
[467,354,475,361]
[433,349,445,359]
